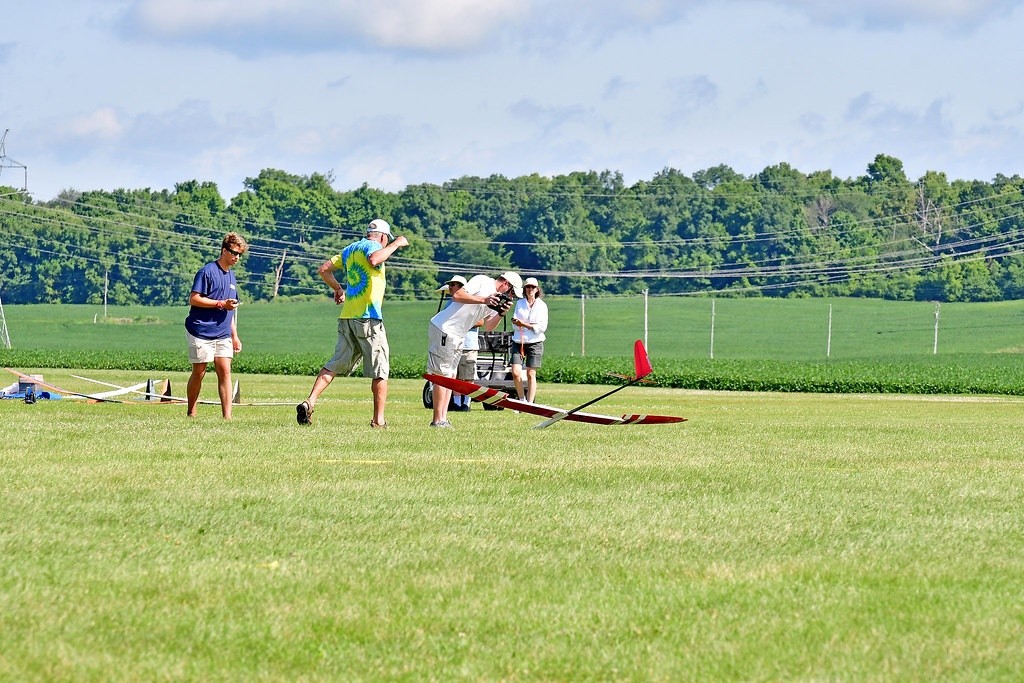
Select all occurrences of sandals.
[370,420,388,429]
[296,400,314,426]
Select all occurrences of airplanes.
[61,374,187,406]
[422,339,689,427]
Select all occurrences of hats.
[523,278,539,287]
[445,274,467,286]
[500,271,523,298]
[366,218,395,240]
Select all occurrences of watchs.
[216,300,224,311]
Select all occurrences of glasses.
[225,247,242,257]
[524,285,536,289]
[446,282,456,286]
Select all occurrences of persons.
[510,277,548,413]
[184,232,249,419]
[427,271,524,426]
[444,275,484,412]
[296,219,409,427]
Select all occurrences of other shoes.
[447,403,461,411]
[458,404,470,411]
[430,421,451,428]
